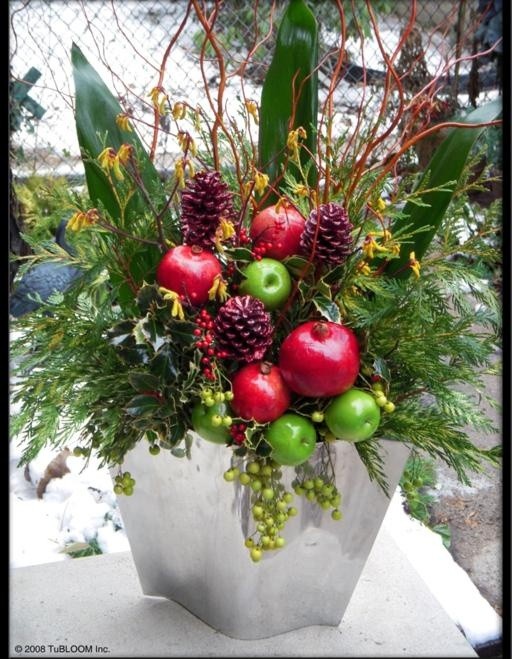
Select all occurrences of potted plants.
[6,2,510,639]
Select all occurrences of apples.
[238,257,292,312]
[191,399,234,444]
[264,414,317,466]
[324,389,381,443]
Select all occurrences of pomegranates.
[228,360,292,424]
[157,244,223,306]
[278,320,360,398]
[250,204,306,261]
